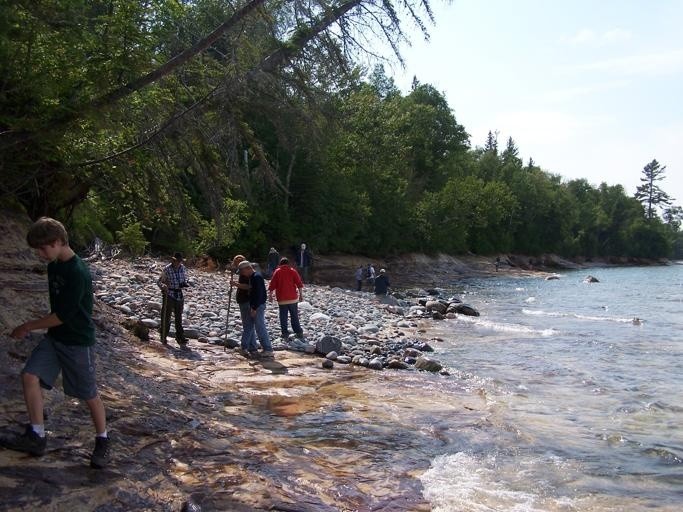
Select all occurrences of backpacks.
[366,267,371,277]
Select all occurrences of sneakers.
[0,424,47,457]
[280,332,303,338]
[91,436,112,467]
[260,351,274,356]
[239,349,249,356]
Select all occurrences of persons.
[267,257,306,338]
[294,243,311,283]
[374,268,389,295]
[228,255,258,356]
[266,248,280,280]
[365,263,375,292]
[235,260,275,358]
[354,265,362,290]
[494,256,501,272]
[0,216,113,469]
[157,251,192,344]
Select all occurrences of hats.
[269,247,277,254]
[231,255,250,268]
[380,269,385,272]
[171,252,186,262]
